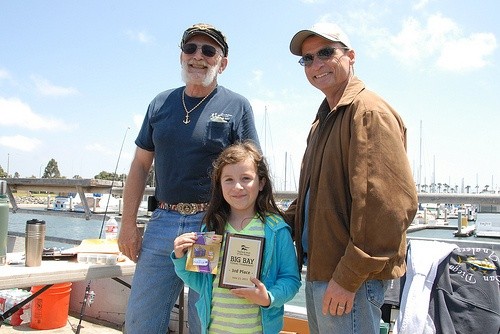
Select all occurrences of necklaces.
[182,85,216,124]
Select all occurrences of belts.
[158,201,210,216]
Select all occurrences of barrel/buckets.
[30,282,72,330]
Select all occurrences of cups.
[25,219,46,267]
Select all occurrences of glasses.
[181,42,224,59]
[297,46,351,66]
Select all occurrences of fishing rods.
[75,126,128,331]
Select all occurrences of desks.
[0,253,137,334]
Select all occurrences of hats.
[289,22,351,56]
[180,22,229,58]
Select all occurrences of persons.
[282,23,419,334]
[169,138,303,334]
[117,23,263,334]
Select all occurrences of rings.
[338,306,346,308]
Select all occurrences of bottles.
[0,289,32,326]
[106,214,118,239]
[0,193,9,266]
